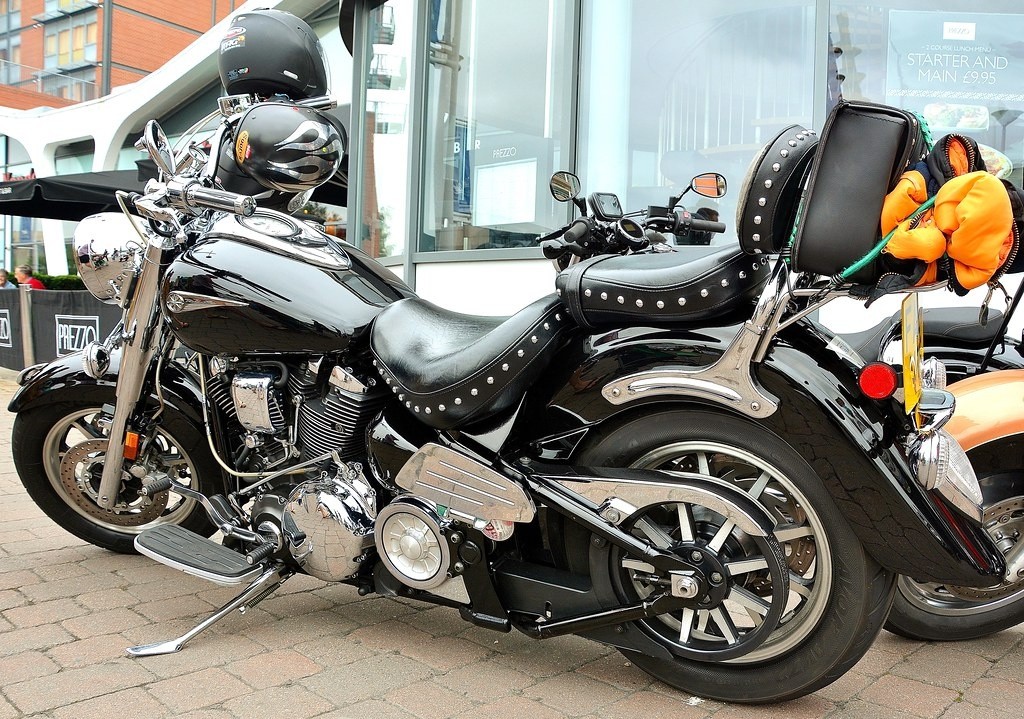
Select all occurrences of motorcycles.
[8,8,1024,709]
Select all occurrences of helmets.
[231,101,347,192]
[218,7,330,101]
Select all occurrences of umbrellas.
[0,167,167,222]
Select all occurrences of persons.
[78,238,129,270]
[13,264,46,290]
[0,269,17,289]
